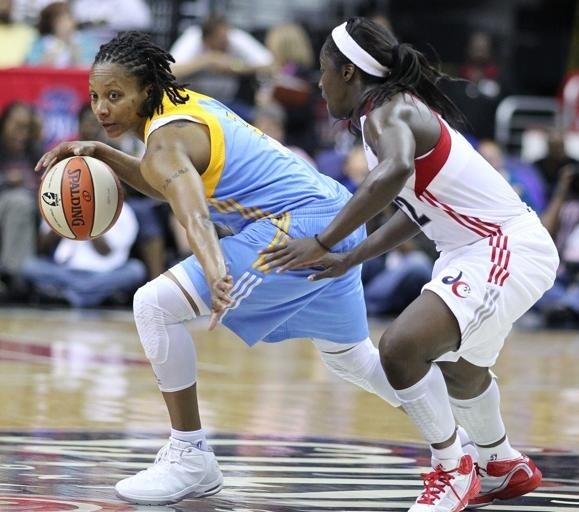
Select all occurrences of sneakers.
[114,436,223,505]
[407,442,543,512]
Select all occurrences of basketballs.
[38,155,124,241]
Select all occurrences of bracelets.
[315,234,331,252]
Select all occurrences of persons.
[35,30,478,506]
[258,15,560,512]
[0,0,579,307]
